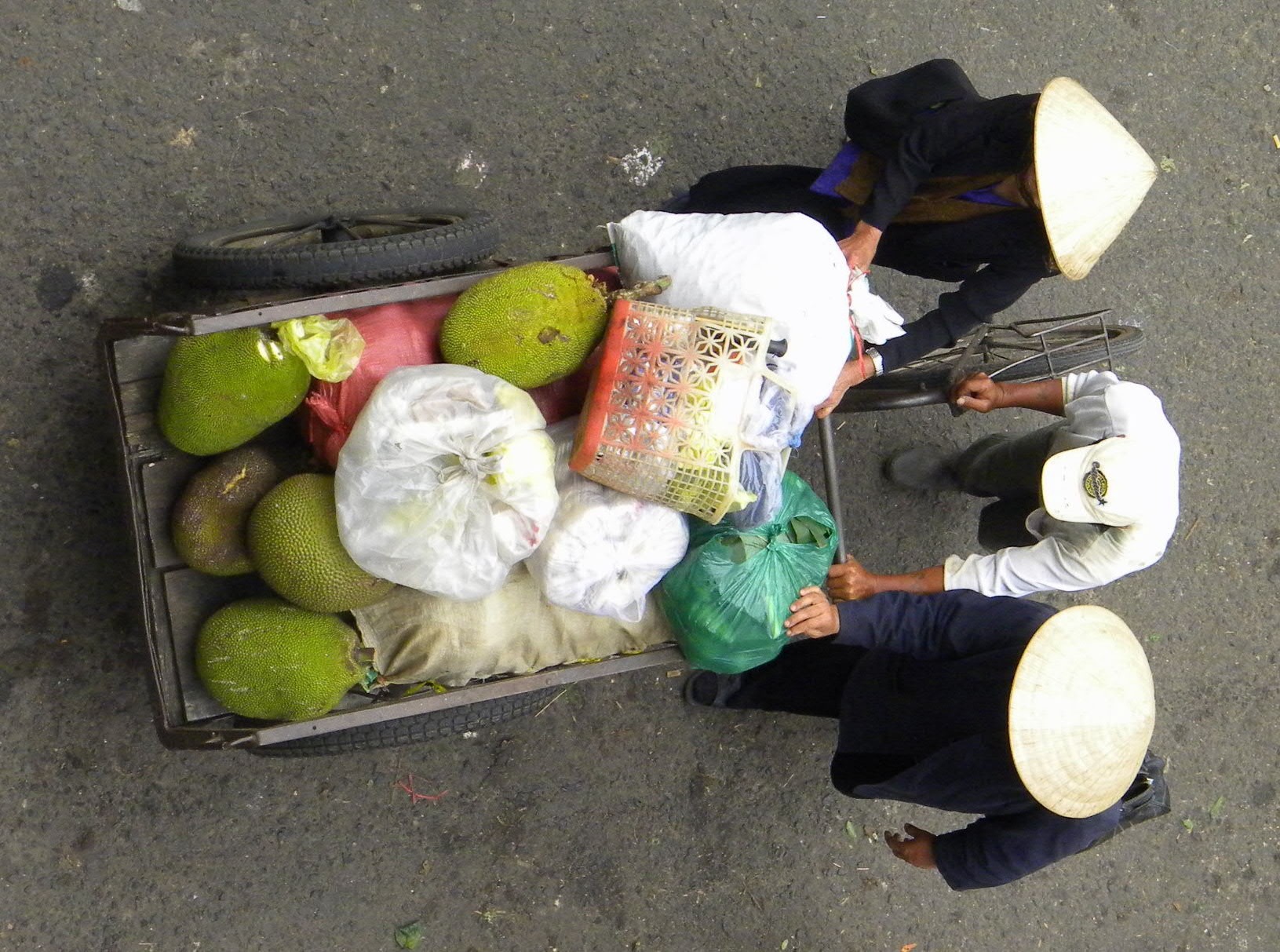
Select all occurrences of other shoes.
[886,447,969,493]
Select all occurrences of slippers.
[684,670,746,709]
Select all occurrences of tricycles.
[99,208,1148,759]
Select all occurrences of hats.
[1041,438,1152,528]
[1033,75,1157,282]
[1010,605,1160,820]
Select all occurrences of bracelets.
[865,347,884,377]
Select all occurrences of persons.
[690,57,1159,420]
[686,587,1159,892]
[829,371,1182,605]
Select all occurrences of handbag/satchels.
[280,301,838,670]
